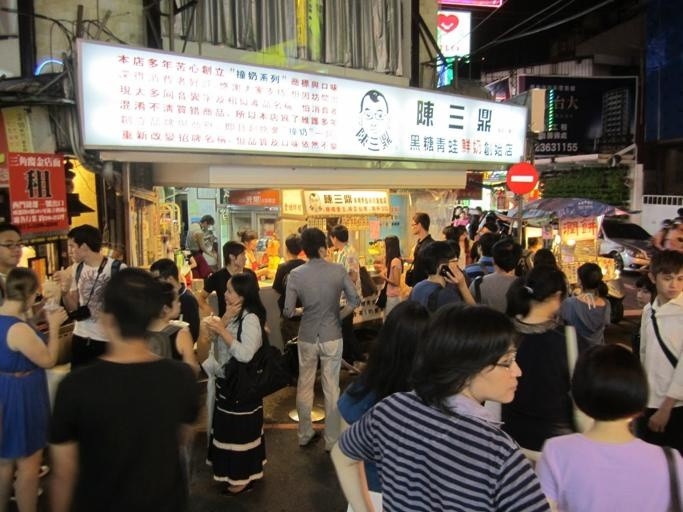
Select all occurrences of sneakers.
[10,487,44,501]
[14,465,49,478]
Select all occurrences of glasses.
[495,351,518,368]
[0,243,24,249]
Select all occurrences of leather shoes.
[216,480,257,497]
[299,430,321,449]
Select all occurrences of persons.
[38,203,681,414]
[535,344,682,512]
[282,225,362,453]
[484,264,594,466]
[337,301,434,512]
[0,224,51,505]
[329,302,550,512]
[145,281,201,493]
[204,275,266,495]
[0,268,70,512]
[48,268,207,512]
[356,89,391,150]
[636,248,683,458]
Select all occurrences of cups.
[38,266,72,316]
[168,320,190,359]
[203,316,219,343]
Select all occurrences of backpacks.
[145,324,180,359]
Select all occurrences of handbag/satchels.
[375,288,387,308]
[223,345,289,402]
[405,270,416,287]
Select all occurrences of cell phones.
[439,265,455,279]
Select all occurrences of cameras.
[70,305,91,321]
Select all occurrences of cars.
[594,219,657,272]
